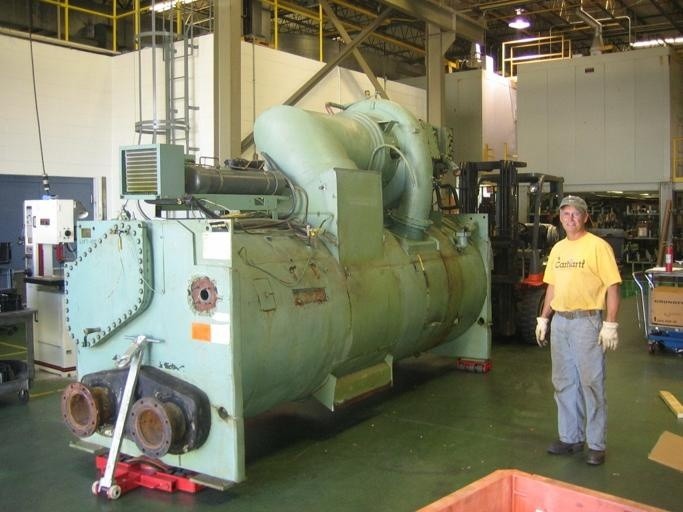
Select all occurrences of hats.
[559,194,587,216]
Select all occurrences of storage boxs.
[647,283,683,328]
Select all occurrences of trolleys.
[631,269,683,355]
[0,304,36,404]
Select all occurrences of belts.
[557,310,597,320]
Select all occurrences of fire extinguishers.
[663,243,673,272]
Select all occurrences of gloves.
[598,321,619,353]
[535,317,550,347]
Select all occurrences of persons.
[534,194,623,465]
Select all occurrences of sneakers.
[546,440,585,454]
[586,448,605,465]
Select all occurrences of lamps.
[505,3,532,31]
